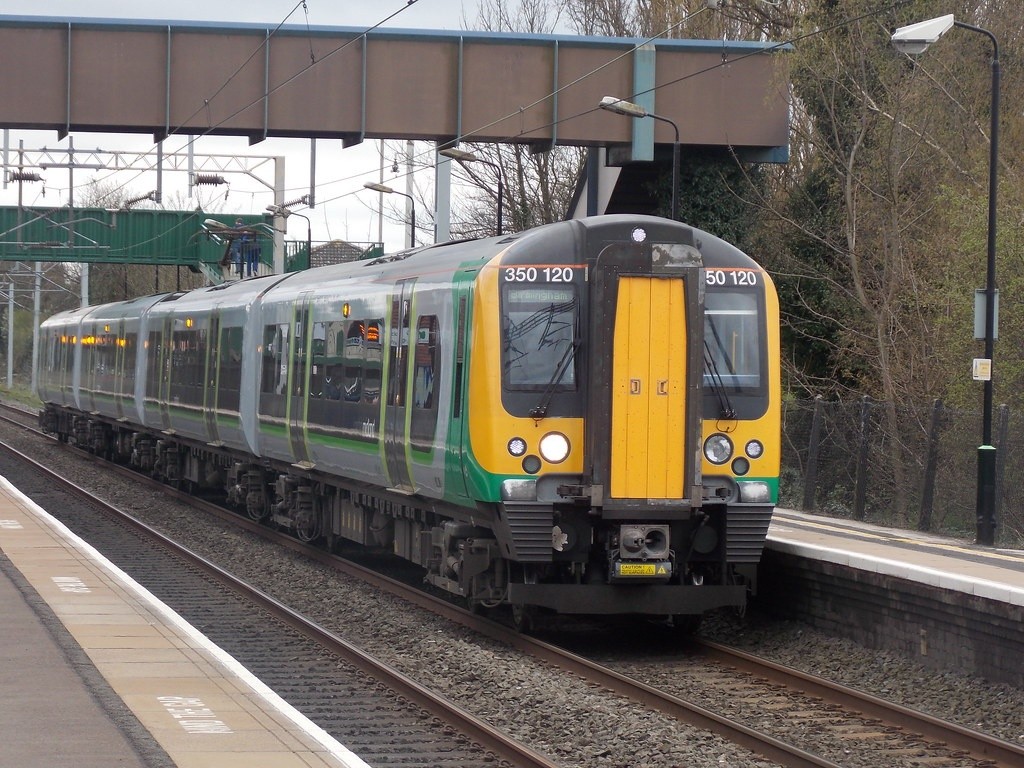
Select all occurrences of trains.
[34,214,779,644]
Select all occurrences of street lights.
[265,204,311,269]
[201,218,244,280]
[439,149,503,236]
[890,12,1001,548]
[597,95,679,221]
[364,181,416,247]
